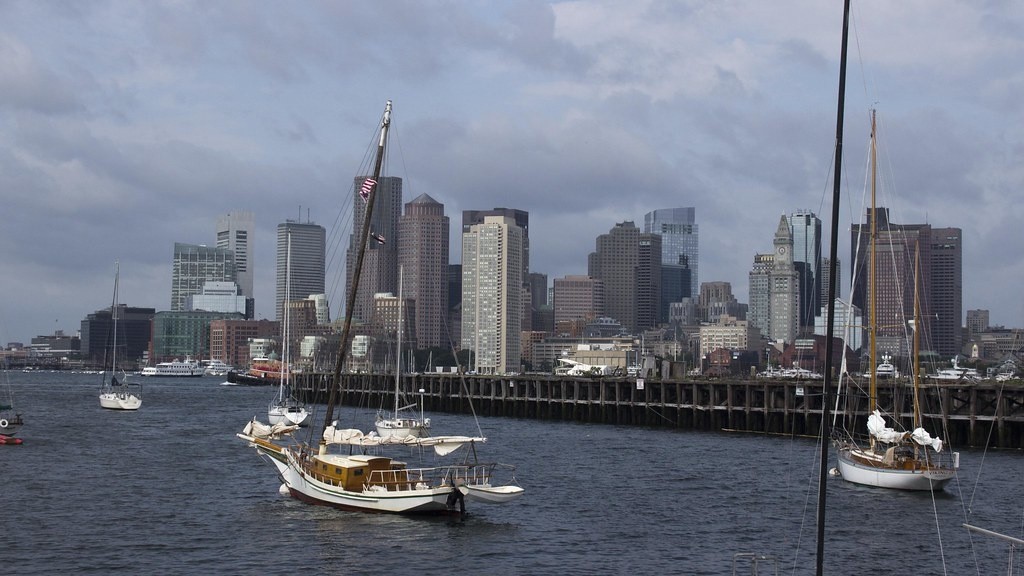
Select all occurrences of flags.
[359,178,379,203]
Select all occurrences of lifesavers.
[0,419,9,428]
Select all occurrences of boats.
[201,358,235,377]
[454,482,523,504]
[875,346,896,378]
[141,354,206,380]
[226,354,297,387]
[918,352,979,382]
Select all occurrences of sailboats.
[0,366,26,445]
[370,263,434,441]
[263,229,315,429]
[98,260,144,412]
[230,99,517,517]
[819,107,960,494]
